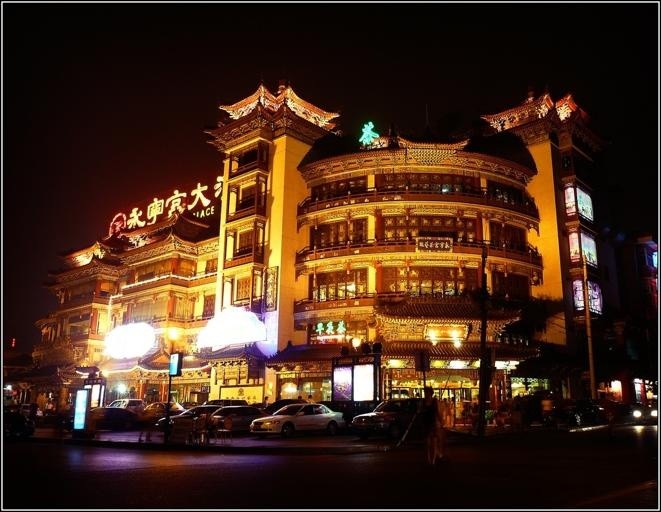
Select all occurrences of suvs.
[350,399,431,442]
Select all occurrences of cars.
[498,389,657,432]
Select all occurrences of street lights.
[168,328,180,402]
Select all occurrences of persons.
[508,396,525,433]
[421,385,443,465]
[262,396,269,409]
[135,404,234,446]
[306,394,316,403]
[298,396,303,401]
